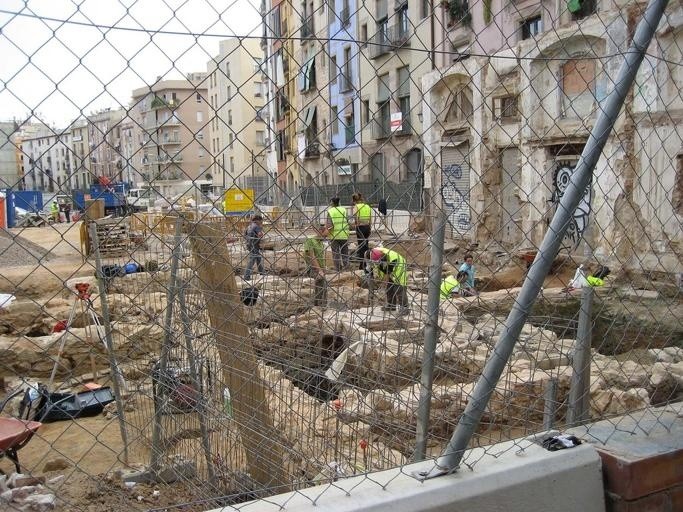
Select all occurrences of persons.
[362,247,411,315]
[51,200,59,225]
[324,195,350,272]
[457,254,475,294]
[438,270,468,302]
[304,225,330,307]
[352,192,372,269]
[240,215,268,281]
[63,203,71,224]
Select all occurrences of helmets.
[370,249,383,261]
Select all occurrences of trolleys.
[0,388,42,480]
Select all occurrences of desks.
[17,213,45,226]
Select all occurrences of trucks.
[0,175,153,218]
